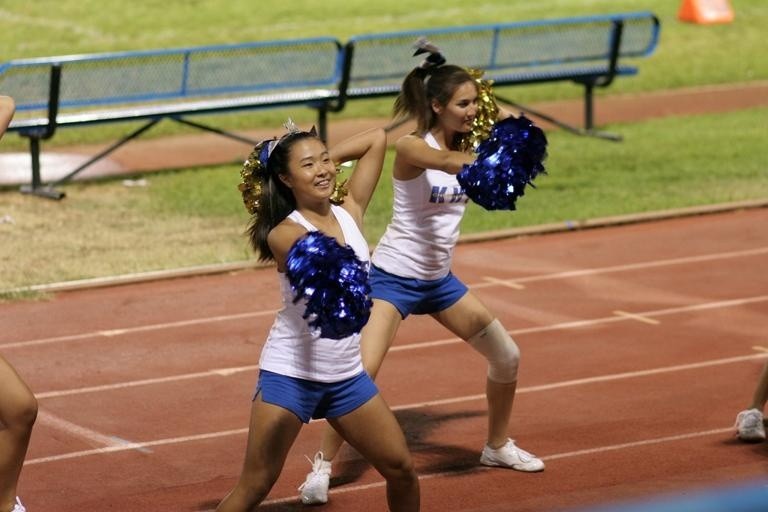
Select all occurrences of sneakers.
[480,439,545,472]
[735,409,764,439]
[301,458,333,504]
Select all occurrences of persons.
[294,39,547,507]
[728,363,767,442]
[208,125,421,512]
[1,91,42,512]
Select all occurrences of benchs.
[0,11,660,201]
